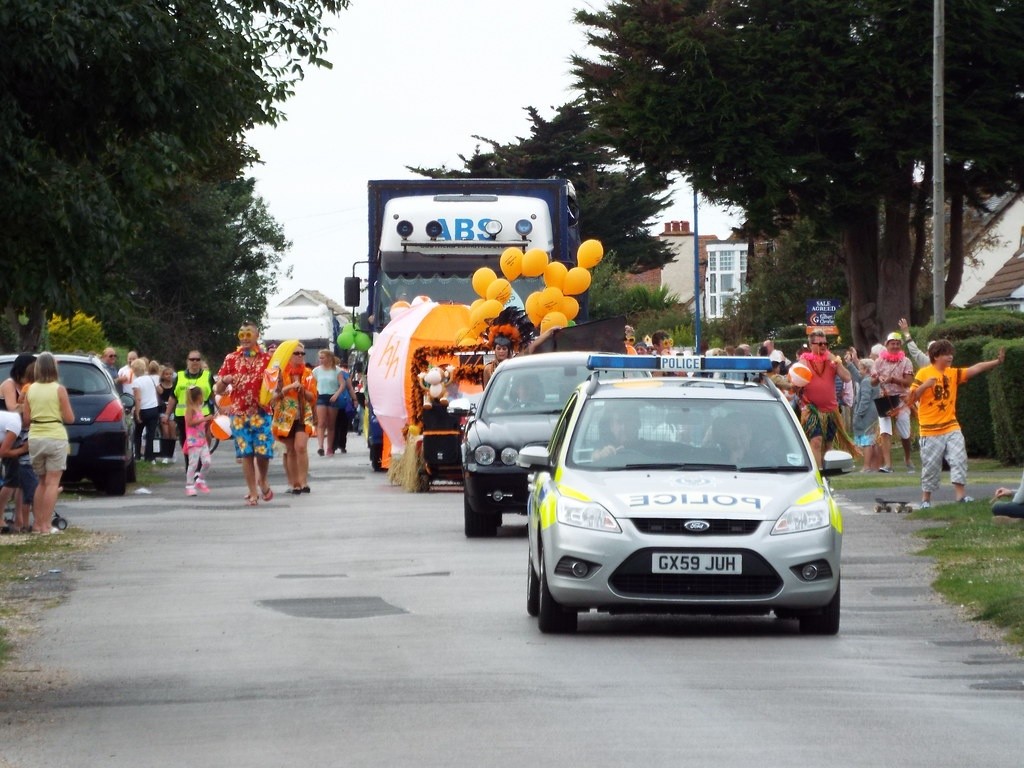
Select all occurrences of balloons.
[390,294,431,321]
[788,363,812,387]
[210,415,233,440]
[452,239,603,347]
[215,393,231,408]
[337,322,371,353]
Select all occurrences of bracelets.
[221,376,228,386]
[905,333,910,338]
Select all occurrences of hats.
[884,332,904,346]
[870,344,884,356]
[927,340,936,350]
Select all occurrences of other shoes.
[921,499,931,509]
[0,523,63,535]
[184,486,197,496]
[907,464,915,473]
[958,496,975,502]
[879,466,894,473]
[136,440,348,494]
[194,479,209,493]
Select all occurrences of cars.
[517,351,855,639]
[0,353,136,498]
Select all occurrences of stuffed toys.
[417,366,455,409]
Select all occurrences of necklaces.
[800,350,829,363]
[879,349,904,362]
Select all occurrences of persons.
[368,284,413,325]
[162,319,365,507]
[0,352,75,536]
[483,326,563,390]
[624,325,915,475]
[73,348,178,465]
[592,408,658,460]
[523,282,538,304]
[700,412,794,467]
[992,469,1024,518]
[898,317,1004,510]
[495,371,542,408]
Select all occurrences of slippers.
[249,494,259,505]
[258,479,273,501]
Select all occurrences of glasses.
[238,331,255,340]
[105,354,117,358]
[293,351,306,356]
[624,336,635,344]
[810,342,828,347]
[189,358,200,361]
[658,338,674,346]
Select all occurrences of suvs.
[447,351,656,539]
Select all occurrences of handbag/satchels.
[872,382,900,418]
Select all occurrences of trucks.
[260,303,356,368]
[344,174,593,476]
